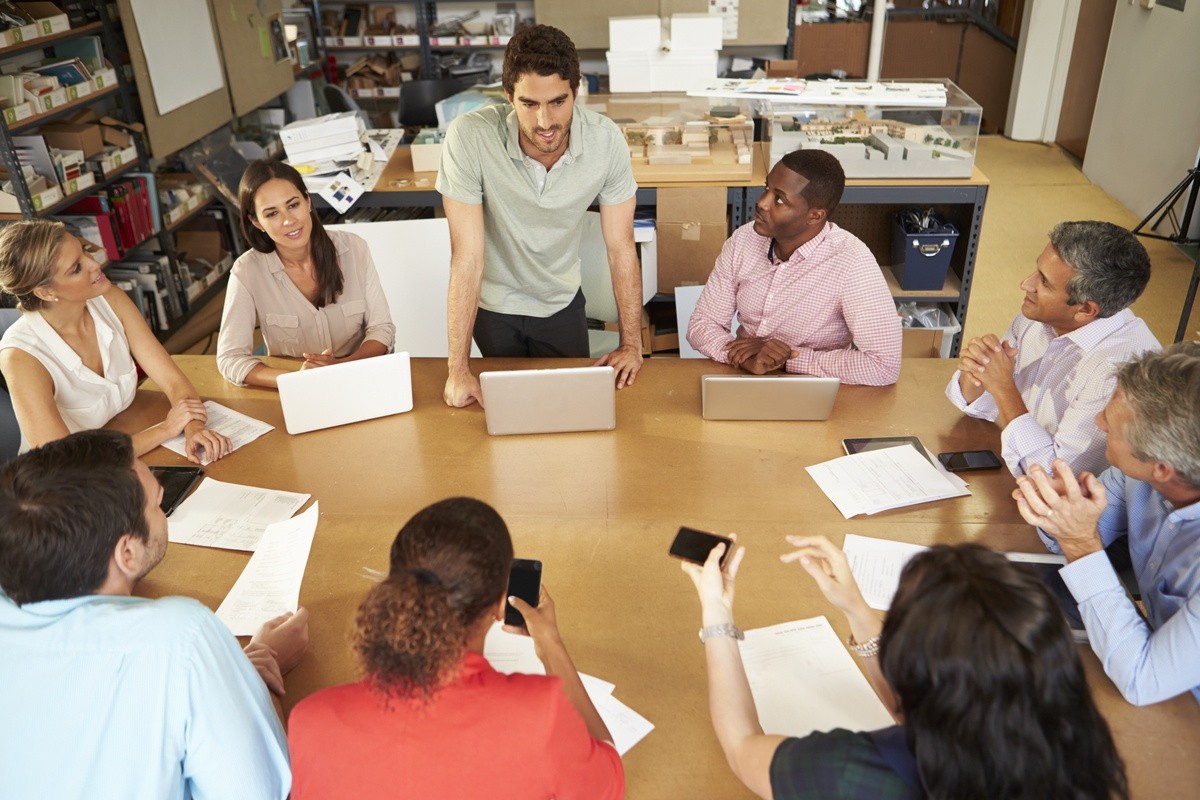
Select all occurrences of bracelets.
[843,626,885,658]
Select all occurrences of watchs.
[696,624,746,643]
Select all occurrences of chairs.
[323,84,375,130]
[396,79,462,144]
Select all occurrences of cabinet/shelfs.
[305,0,522,142]
[0,0,246,362]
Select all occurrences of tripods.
[1131,147,1200,249]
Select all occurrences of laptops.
[276,350,414,435]
[701,372,840,422]
[479,366,616,436]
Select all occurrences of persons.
[286,497,629,800]
[684,147,905,388]
[1009,335,1200,714]
[944,219,1163,495]
[216,158,396,389]
[435,24,644,409]
[1,429,315,800]
[678,527,1134,800]
[0,220,233,469]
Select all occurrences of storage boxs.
[321,33,518,99]
[279,108,369,166]
[410,128,443,173]
[898,299,961,358]
[151,171,233,302]
[761,77,982,179]
[574,92,756,183]
[0,0,145,216]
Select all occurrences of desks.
[277,142,992,356]
[96,356,1190,800]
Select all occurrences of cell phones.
[669,526,733,570]
[502,558,543,636]
[939,449,1002,472]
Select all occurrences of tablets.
[842,436,938,472]
[148,464,202,517]
[1000,549,1090,645]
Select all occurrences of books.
[56,174,193,341]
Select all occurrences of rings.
[1046,509,1053,518]
